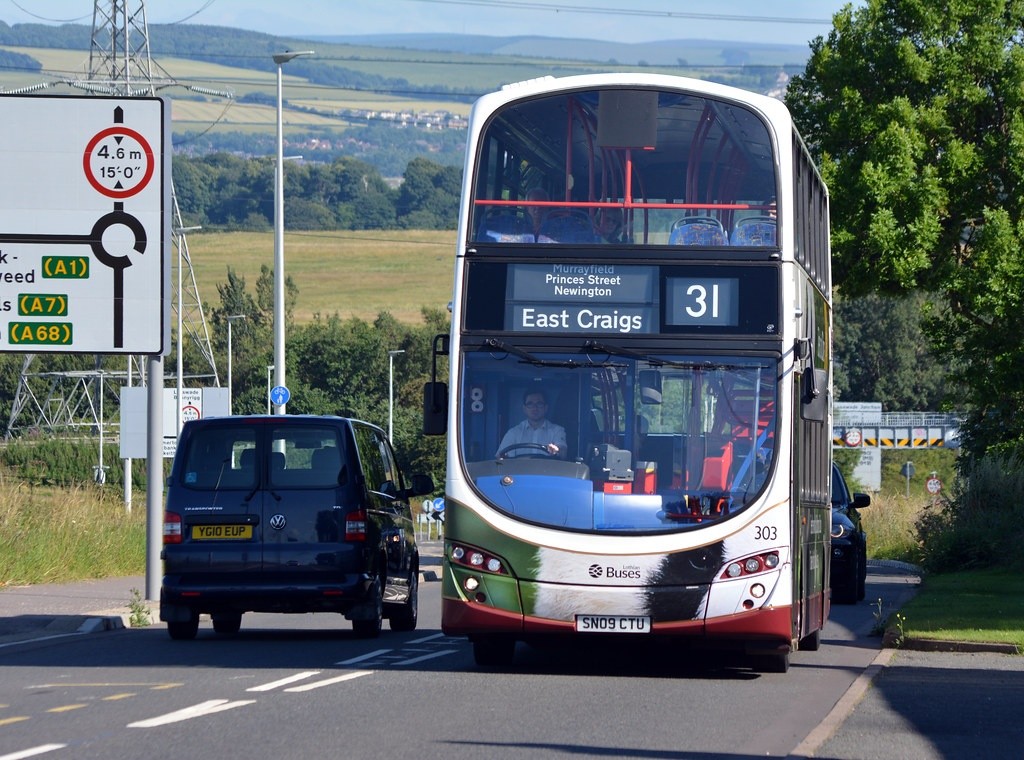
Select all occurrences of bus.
[439,71,834,674]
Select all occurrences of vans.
[159,414,422,640]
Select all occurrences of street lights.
[271,50,315,414]
[175,225,204,446]
[387,350,405,444]
[267,365,276,415]
[135,83,233,603]
[225,314,247,416]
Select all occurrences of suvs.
[829,462,872,603]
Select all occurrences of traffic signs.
[0,91,173,356]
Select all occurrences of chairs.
[169,444,401,541]
[457,215,779,526]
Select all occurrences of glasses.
[524,401,548,408]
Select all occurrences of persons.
[494,387,568,461]
